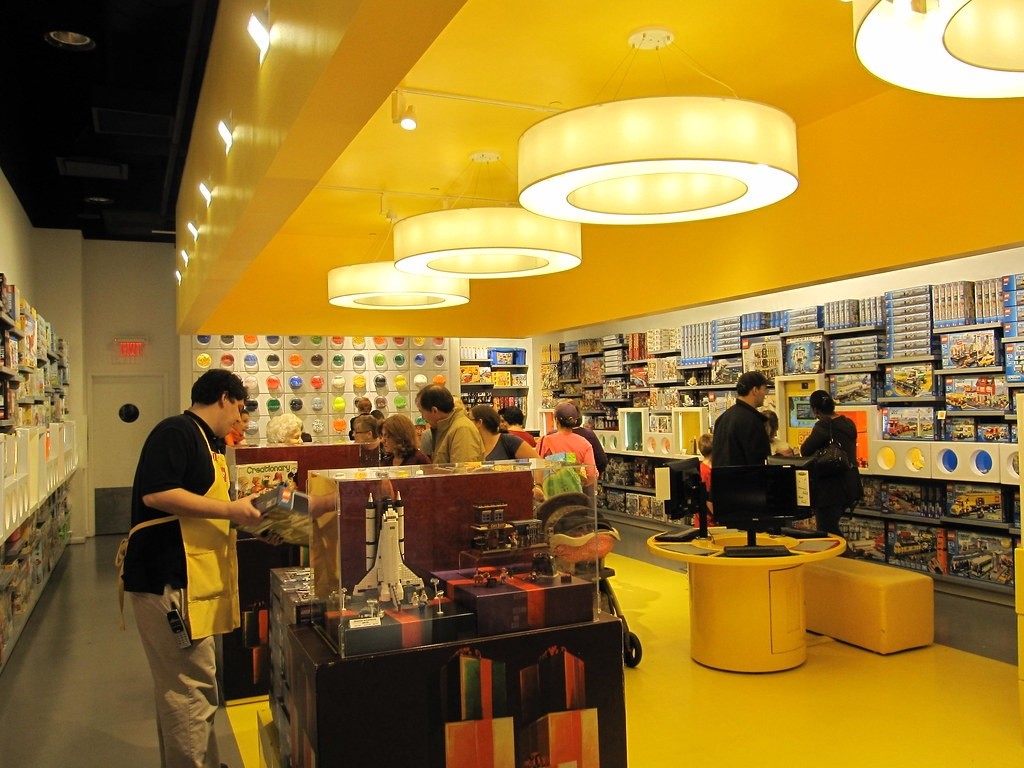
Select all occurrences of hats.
[554,402,578,424]
[737,370,774,388]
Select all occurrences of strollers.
[536,492,642,668]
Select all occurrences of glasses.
[467,416,486,423]
[380,432,396,441]
[352,430,373,434]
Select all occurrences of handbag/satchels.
[811,419,849,471]
[541,436,583,502]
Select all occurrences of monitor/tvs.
[663,457,708,538]
[711,455,821,547]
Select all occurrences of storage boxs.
[326,587,475,659]
[886,521,948,575]
[430,560,596,638]
[977,423,1010,443]
[783,341,825,374]
[829,360,876,369]
[883,407,941,441]
[830,373,877,405]
[741,335,786,385]
[946,481,1010,522]
[886,362,939,397]
[829,335,886,347]
[829,343,886,355]
[487,347,515,365]
[492,371,511,387]
[881,484,944,519]
[511,373,527,387]
[829,351,885,362]
[945,425,977,442]
[940,330,1001,369]
[947,528,1014,587]
[838,515,886,563]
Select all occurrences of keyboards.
[779,526,829,540]
[654,526,699,542]
[724,544,791,557]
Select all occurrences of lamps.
[391,91,417,131]
[327,207,471,311]
[187,14,269,242]
[392,151,583,280]
[516,28,798,225]
[851,0,1024,100]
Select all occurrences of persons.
[710,371,794,532]
[498,406,537,447]
[799,390,863,556]
[541,400,609,475]
[416,383,484,464]
[380,414,431,467]
[347,398,386,468]
[226,409,249,446]
[541,402,599,488]
[694,433,717,527]
[761,410,789,454]
[113,368,263,768]
[469,406,540,461]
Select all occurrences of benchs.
[801,555,935,655]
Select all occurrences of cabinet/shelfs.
[0,272,79,676]
[460,272,1024,609]
